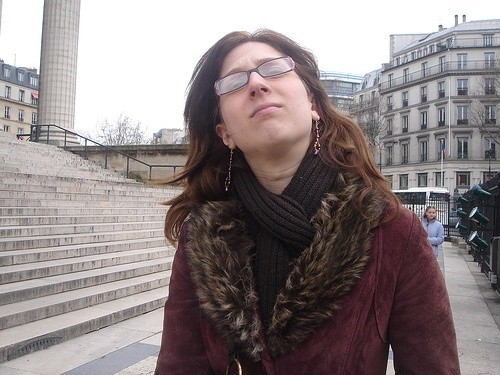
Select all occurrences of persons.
[154,28,460,375]
[420,206,444,258]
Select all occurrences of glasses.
[213,55,295,95]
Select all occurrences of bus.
[392,187,451,227]
